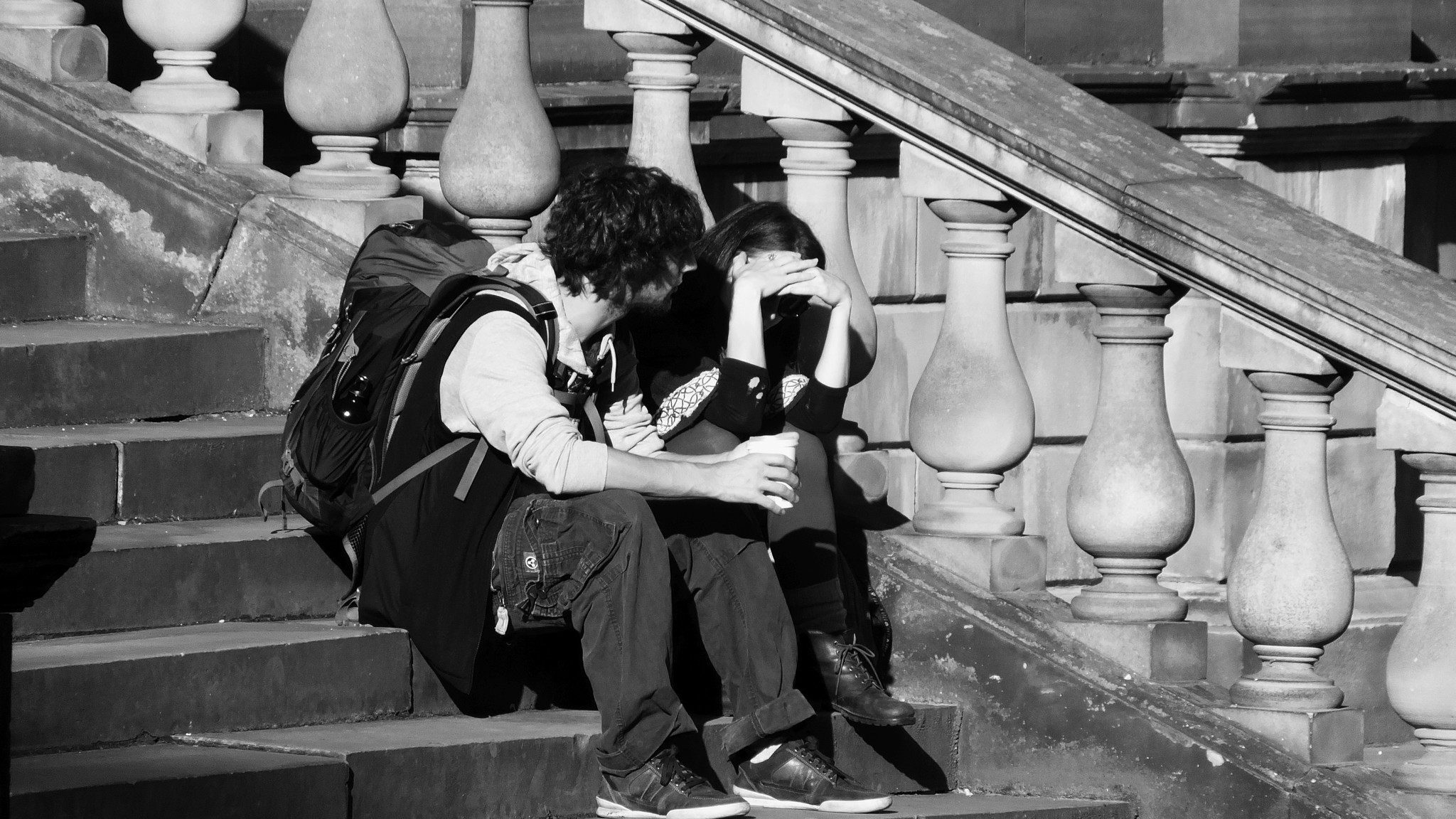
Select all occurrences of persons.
[636,201,918,727]
[361,161,895,819]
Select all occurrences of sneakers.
[731,745,894,814]
[595,738,750,819]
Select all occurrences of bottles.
[337,373,370,424]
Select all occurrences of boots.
[791,629,920,729]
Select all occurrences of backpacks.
[258,217,606,625]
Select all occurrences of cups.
[745,439,798,508]
